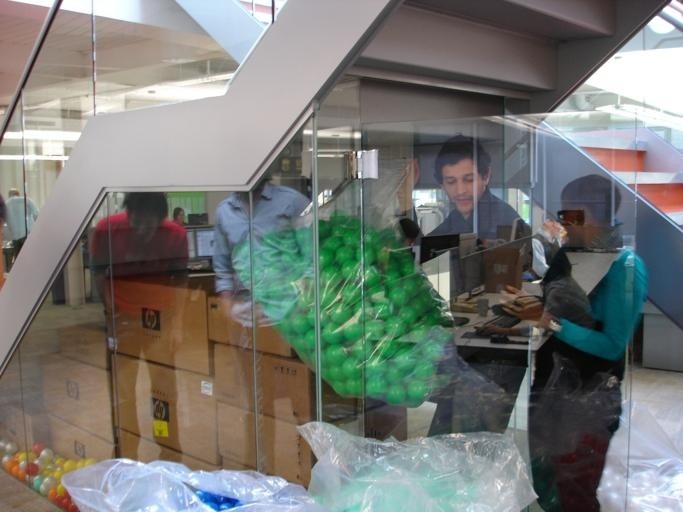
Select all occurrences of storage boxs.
[6,270,409,489]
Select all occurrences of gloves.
[259,298,291,328]
[232,300,257,329]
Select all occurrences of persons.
[0,194,7,289]
[392,129,539,442]
[6,186,40,261]
[91,189,191,459]
[500,174,649,511]
[171,206,187,225]
[214,170,314,493]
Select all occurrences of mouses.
[490,333,509,343]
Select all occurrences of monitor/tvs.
[188,213,208,225]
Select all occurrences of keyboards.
[476,313,521,336]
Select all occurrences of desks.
[398,252,624,351]
